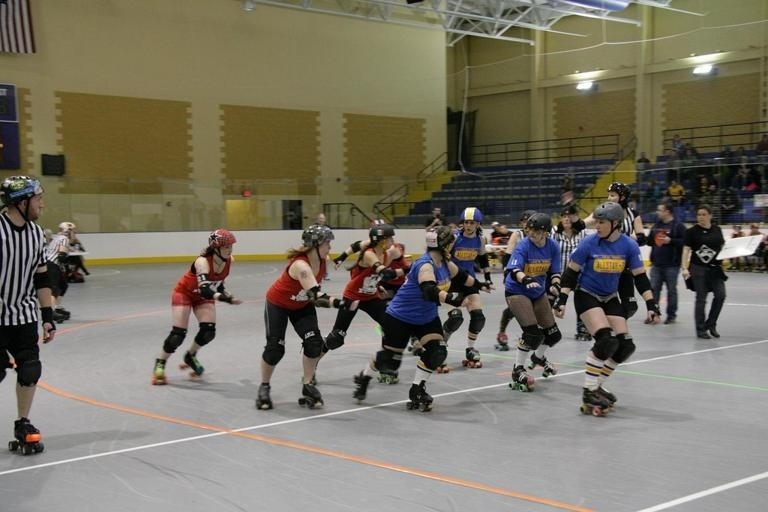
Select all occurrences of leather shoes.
[705,319,720,337]
[697,331,710,339]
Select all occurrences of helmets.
[302,224,334,246]
[0,176,44,203]
[527,213,551,231]
[520,210,536,221]
[593,202,624,221]
[460,207,483,222]
[369,219,394,239]
[58,221,75,238]
[607,182,631,198]
[208,228,235,249]
[428,226,455,251]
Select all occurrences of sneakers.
[664,318,675,324]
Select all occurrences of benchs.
[387,140,768,227]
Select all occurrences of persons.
[46,222,82,321]
[69,230,90,275]
[333,220,424,355]
[257,223,360,400]
[354,226,496,405]
[314,223,412,377]
[314,214,329,280]
[154,229,242,380]
[0,174,57,444]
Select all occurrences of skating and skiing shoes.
[9,418,44,454]
[495,334,510,351]
[298,382,323,409]
[354,370,372,404]
[509,363,534,392]
[376,368,399,384]
[180,351,204,377]
[51,306,70,324]
[406,379,433,412]
[256,384,272,410]
[574,322,592,341]
[407,337,423,355]
[437,360,449,373]
[580,384,617,416]
[152,358,168,384]
[462,347,483,368]
[528,350,556,377]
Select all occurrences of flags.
[0,0,37,55]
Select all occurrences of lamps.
[575,80,598,92]
[692,64,713,75]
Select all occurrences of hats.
[561,207,570,216]
